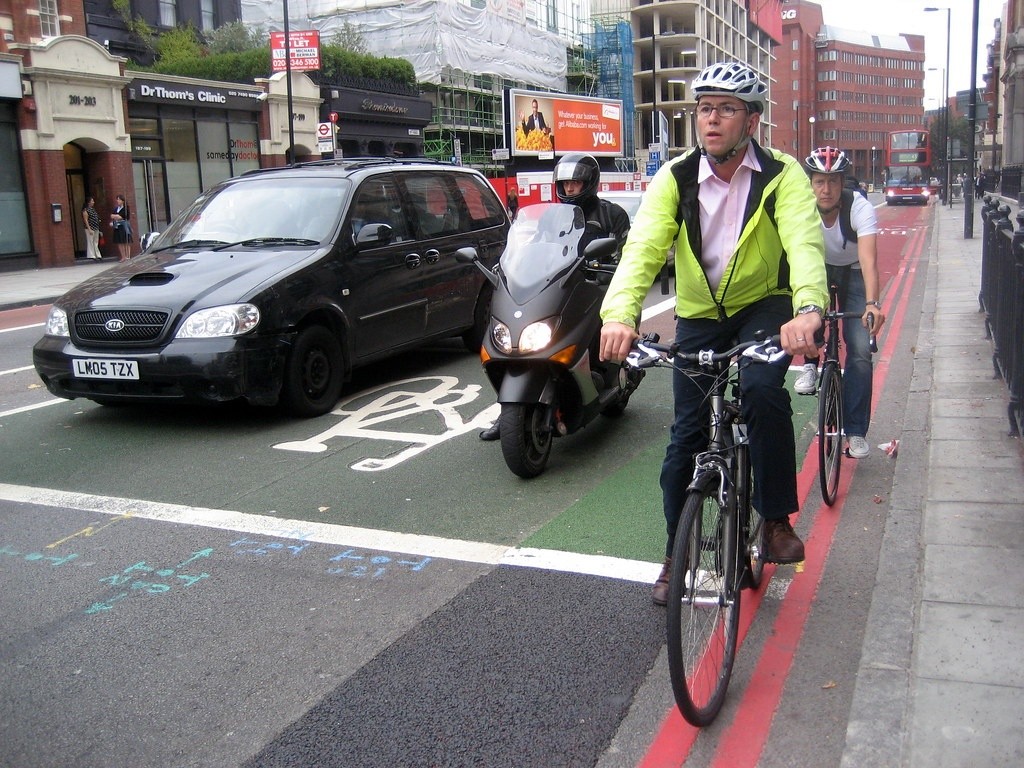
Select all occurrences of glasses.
[696,103,753,118]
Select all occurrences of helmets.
[552,153,600,207]
[803,146,853,174]
[691,63,769,103]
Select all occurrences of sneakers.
[794,363,818,393]
[846,434,870,458]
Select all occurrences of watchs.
[798,305,822,316]
[865,300,881,310]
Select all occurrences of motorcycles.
[454,203,645,481]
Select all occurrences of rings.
[796,338,805,342]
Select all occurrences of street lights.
[652,31,678,140]
[872,146,875,194]
[808,118,815,153]
[503,163,515,209]
[928,67,947,206]
[924,7,951,205]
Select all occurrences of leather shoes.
[480,414,502,440]
[761,517,805,562]
[652,558,686,605]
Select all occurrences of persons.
[793,146,885,459]
[110,194,134,262]
[520,99,547,137]
[957,172,985,199]
[508,188,518,222]
[599,62,832,606]
[478,152,631,440]
[81,196,103,260]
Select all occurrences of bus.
[886,130,932,206]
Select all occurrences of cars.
[930,176,945,196]
[882,181,886,193]
[597,190,676,279]
[33,158,512,420]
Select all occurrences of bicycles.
[622,334,828,729]
[802,281,877,506]
